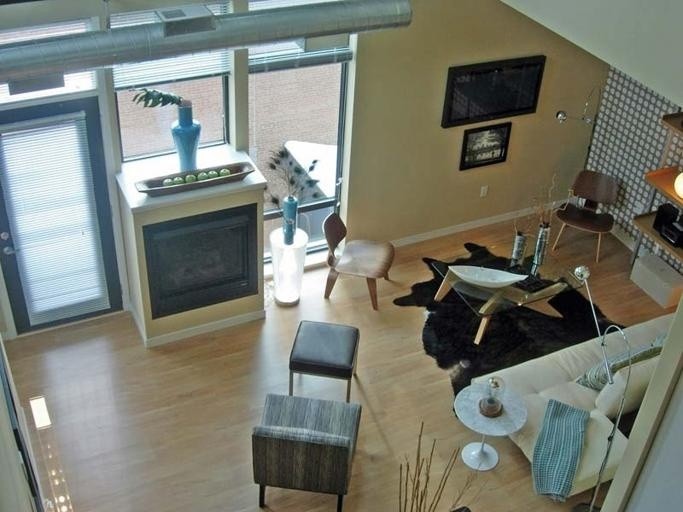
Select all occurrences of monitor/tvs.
[440,55,547,129]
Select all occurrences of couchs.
[471,312,676,497]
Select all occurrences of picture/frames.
[460,123,512,171]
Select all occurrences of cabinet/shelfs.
[629,112,683,267]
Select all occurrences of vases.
[283,195,298,235]
[171,99,201,172]
[283,219,294,245]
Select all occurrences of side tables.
[454,386,528,471]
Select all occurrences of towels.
[530,398,591,504]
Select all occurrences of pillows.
[650,333,666,345]
[573,346,662,390]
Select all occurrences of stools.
[289,320,360,402]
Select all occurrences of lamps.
[29,396,72,511]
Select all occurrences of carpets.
[394,242,628,397]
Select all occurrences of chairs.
[553,171,618,262]
[252,393,363,511]
[322,212,395,311]
